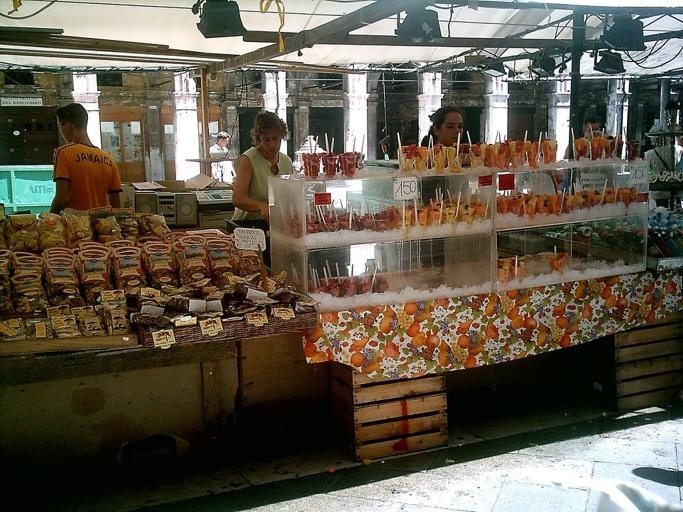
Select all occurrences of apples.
[303,279,677,375]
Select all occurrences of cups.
[310,274,391,300]
[303,150,361,178]
[395,141,556,172]
[493,256,567,283]
[496,186,651,226]
[572,136,641,163]
[289,195,484,232]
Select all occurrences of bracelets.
[464,154,469,167]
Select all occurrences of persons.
[417,106,484,269]
[209,131,237,184]
[560,117,605,197]
[230,110,292,224]
[641,122,683,211]
[49,103,123,216]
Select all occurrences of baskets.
[135,308,319,349]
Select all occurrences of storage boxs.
[119,174,215,226]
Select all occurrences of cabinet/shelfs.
[267,159,683,461]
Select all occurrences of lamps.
[528,51,556,79]
[197,0,249,38]
[481,55,508,78]
[593,49,626,74]
[600,12,647,51]
[395,5,442,42]
